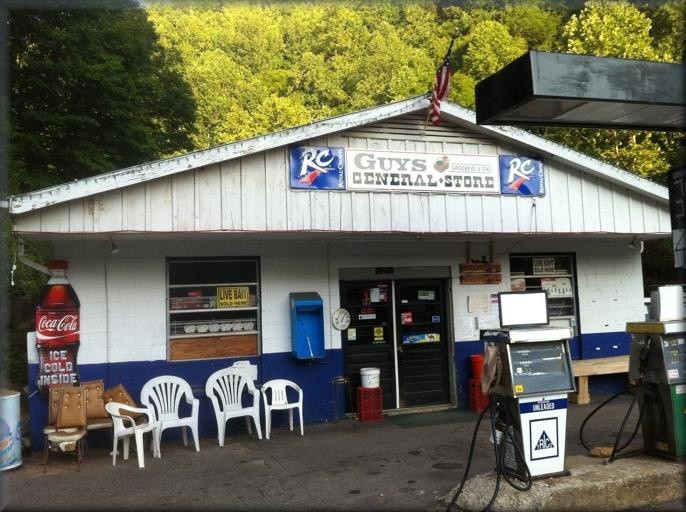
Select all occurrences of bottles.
[35,258,80,398]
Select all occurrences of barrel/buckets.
[360,367,380,389]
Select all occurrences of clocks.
[333,308,350,331]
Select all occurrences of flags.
[429,39,455,127]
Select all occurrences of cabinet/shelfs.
[170,282,257,339]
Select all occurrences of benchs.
[568,355,629,405]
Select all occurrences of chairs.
[42,375,200,474]
[206,366,263,447]
[261,379,304,440]
